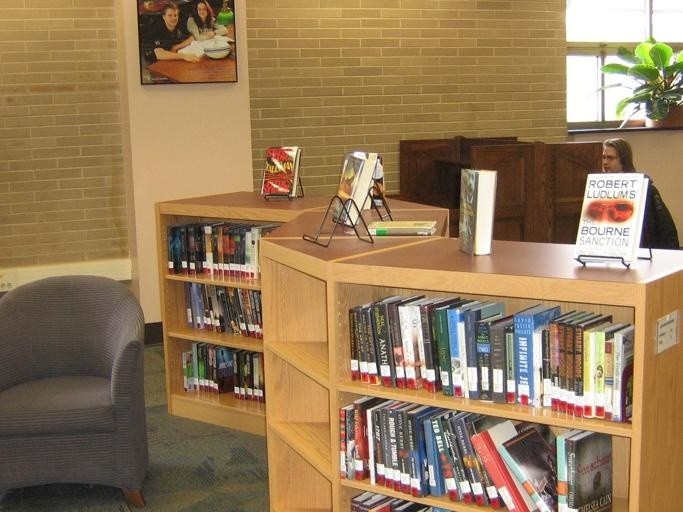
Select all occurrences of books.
[340,396,612,511]
[572,172,650,264]
[332,152,385,228]
[458,168,498,258]
[260,146,302,198]
[366,220,438,237]
[350,294,634,423]
[166,221,282,404]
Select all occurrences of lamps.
[217,0,234,25]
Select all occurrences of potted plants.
[595,37,683,129]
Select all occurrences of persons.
[601,137,679,250]
[186,0,216,42]
[143,2,200,64]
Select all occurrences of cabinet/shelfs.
[328,235,683,511]
[258,235,443,512]
[156,190,338,437]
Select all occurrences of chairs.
[1,274,146,508]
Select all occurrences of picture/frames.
[137,1,238,86]
